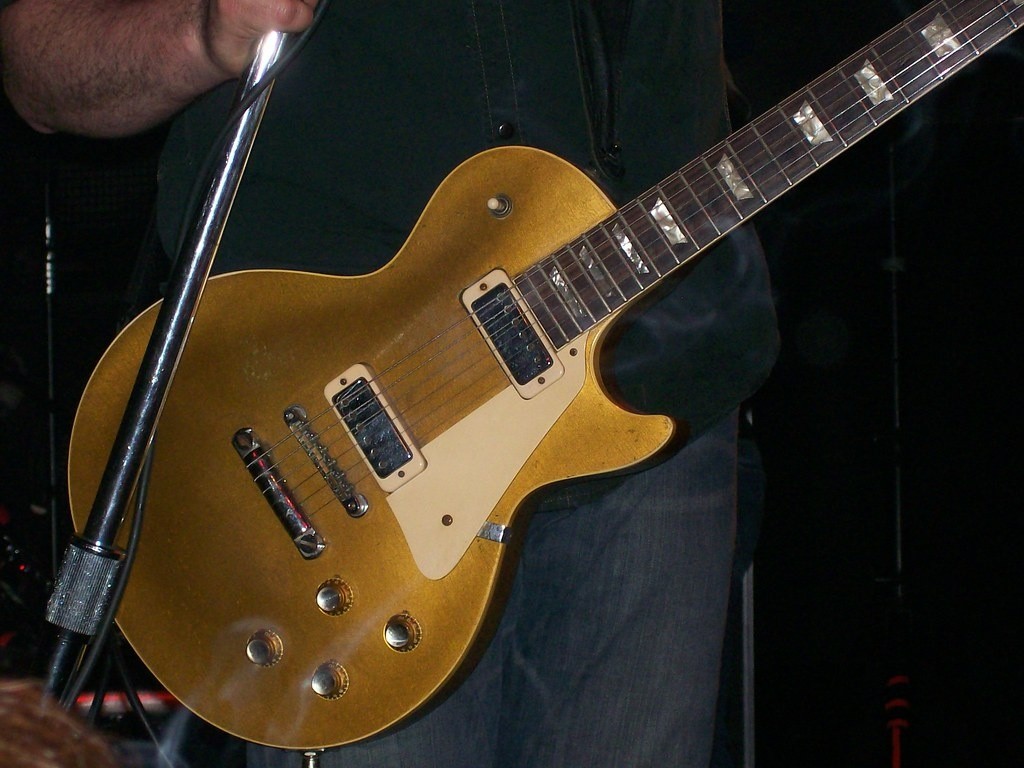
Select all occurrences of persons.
[0,1,778,768]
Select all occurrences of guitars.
[67,0,1023,752]
